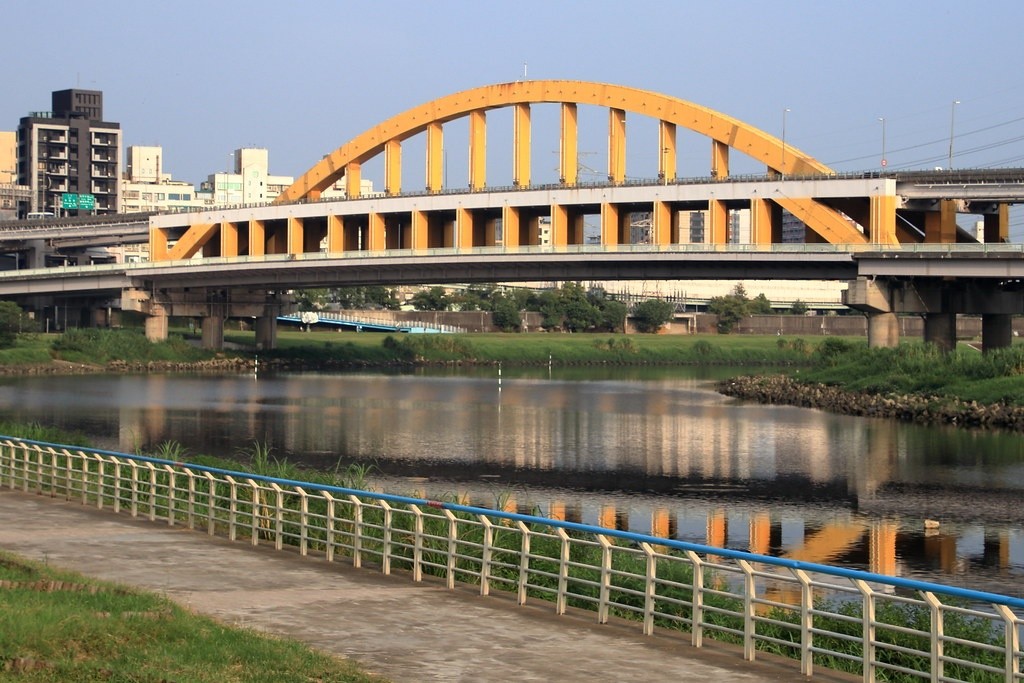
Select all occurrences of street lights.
[226,153,235,206]
[949,100,961,169]
[877,116,887,170]
[781,109,791,181]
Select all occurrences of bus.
[27,212,55,222]
